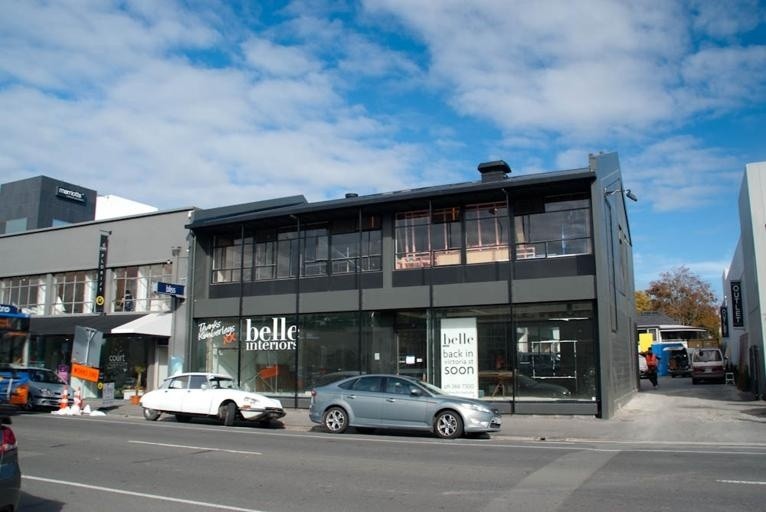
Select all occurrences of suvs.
[0,363,74,408]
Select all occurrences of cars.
[0,422,21,511]
[308,374,502,439]
[638,354,647,379]
[476,371,570,399]
[139,372,285,426]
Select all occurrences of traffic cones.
[59,386,69,410]
[72,387,82,409]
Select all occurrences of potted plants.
[131,382,142,405]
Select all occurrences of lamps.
[606,188,637,202]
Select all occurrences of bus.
[636,325,717,355]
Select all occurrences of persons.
[120,290,134,311]
[639,347,660,388]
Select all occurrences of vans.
[690,349,728,385]
[663,346,695,378]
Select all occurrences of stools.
[725,372,736,387]
